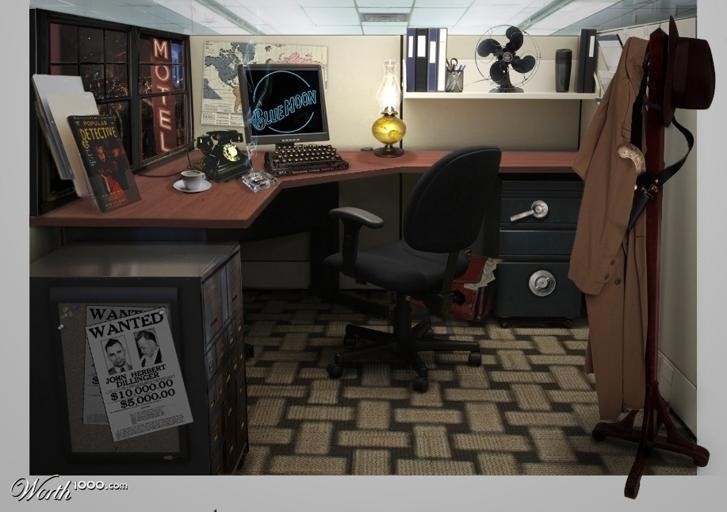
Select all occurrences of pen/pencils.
[445,65,466,92]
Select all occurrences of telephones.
[197,130,252,182]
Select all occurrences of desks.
[38,146,589,326]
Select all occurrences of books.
[66,114,145,215]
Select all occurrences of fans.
[477,23,541,92]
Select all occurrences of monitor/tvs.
[240,62,330,148]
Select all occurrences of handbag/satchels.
[651,14,716,111]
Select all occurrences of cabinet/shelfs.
[33,241,252,474]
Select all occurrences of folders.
[577,28,597,92]
[407,28,447,91]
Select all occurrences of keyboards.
[264,145,349,176]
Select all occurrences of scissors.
[446,58,458,72]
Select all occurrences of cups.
[555,48,572,93]
[181,170,205,190]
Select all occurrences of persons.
[134,330,163,367]
[105,338,133,374]
[91,142,111,176]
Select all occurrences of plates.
[173,179,211,193]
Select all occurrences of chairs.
[326,147,504,392]
